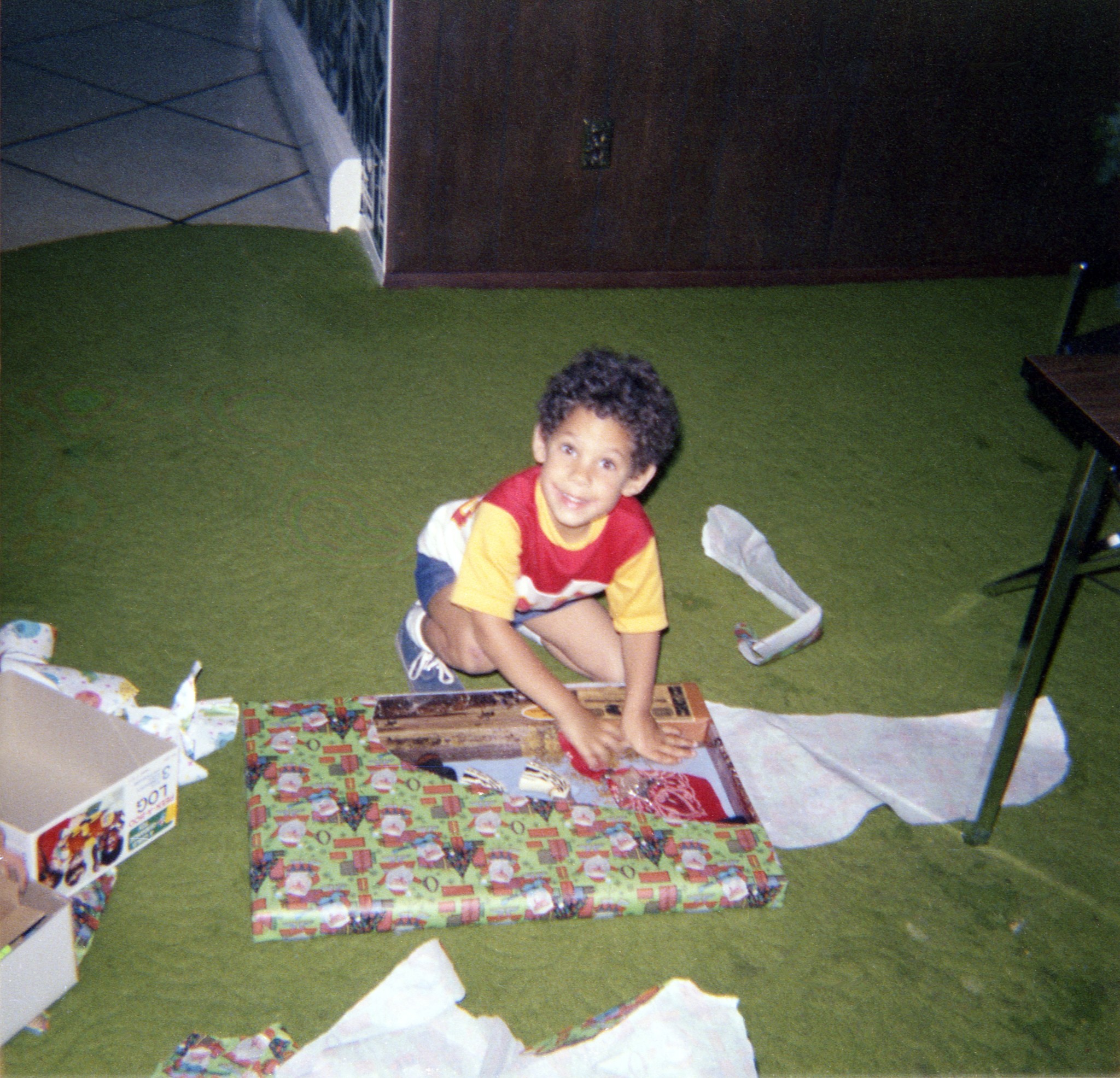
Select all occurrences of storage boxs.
[1,870,82,1045]
[0,668,179,900]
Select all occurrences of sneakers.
[393,605,467,692]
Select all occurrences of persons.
[394,345,699,774]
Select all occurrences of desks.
[958,344,1120,849]
